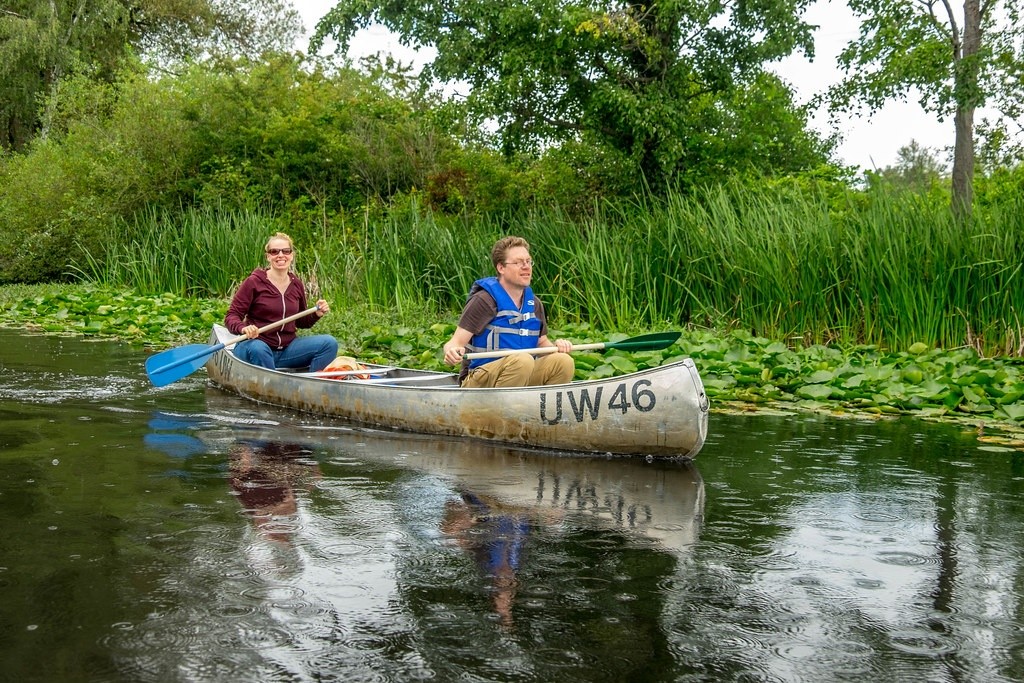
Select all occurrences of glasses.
[268,249,293,255]
[502,260,536,267]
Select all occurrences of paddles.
[457,330,683,360]
[143,304,321,389]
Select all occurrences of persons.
[442,236,575,389]
[223,232,339,372]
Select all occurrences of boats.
[201,322,711,460]
[208,393,707,553]
